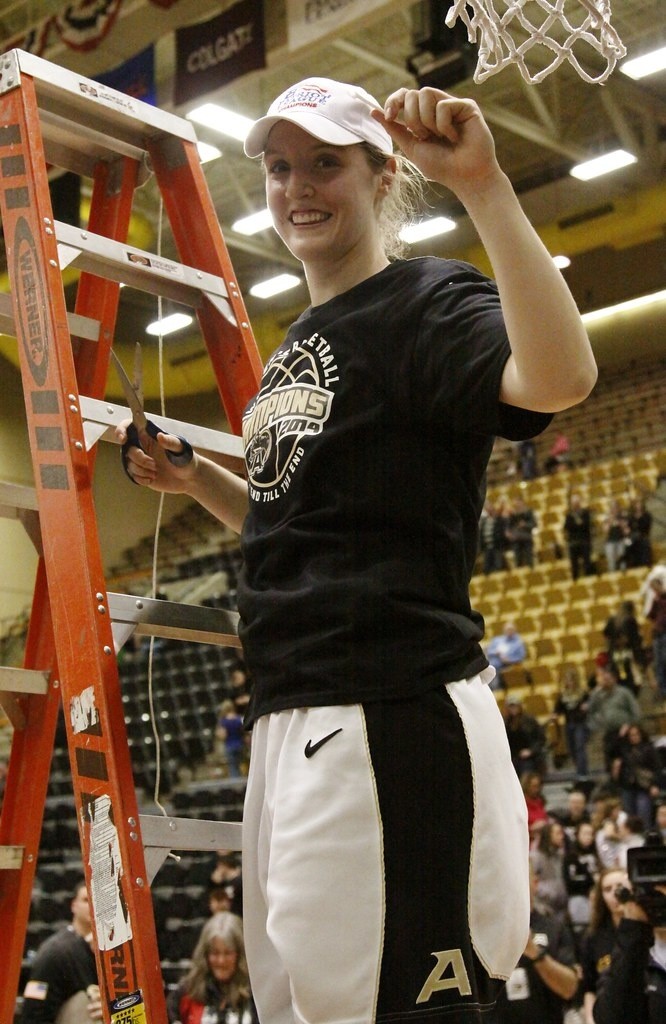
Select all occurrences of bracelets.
[531,950,548,965]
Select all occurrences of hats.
[245,77,393,157]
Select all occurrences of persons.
[118,78,598,1024]
[22,698,257,1024]
[473,421,666,1024]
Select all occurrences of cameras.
[616,844,666,927]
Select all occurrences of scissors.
[109,347,193,485]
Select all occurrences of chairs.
[11,353,666,1024]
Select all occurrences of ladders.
[2,46,268,1024]
